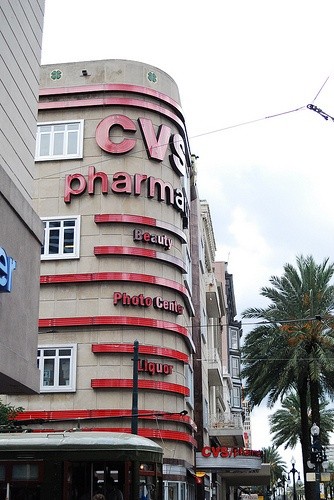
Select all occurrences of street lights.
[276,475,289,500]
[307,423,330,500]
[287,456,301,500]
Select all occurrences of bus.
[0,431,160,500]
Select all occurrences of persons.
[95,477,124,500]
